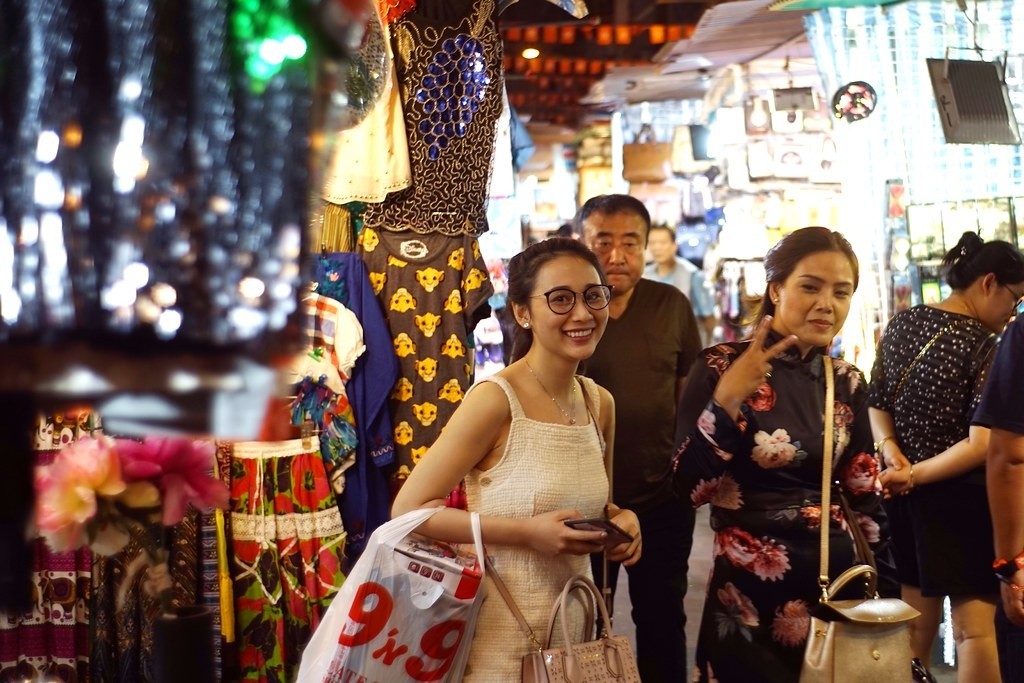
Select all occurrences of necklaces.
[521,359,576,425]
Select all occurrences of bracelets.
[994,552,1024,582]
[874,436,896,451]
[910,465,913,487]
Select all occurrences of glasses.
[528,283,612,315]
[1002,283,1024,312]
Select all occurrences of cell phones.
[562,518,634,546]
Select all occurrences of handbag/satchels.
[520,573,642,683]
[297,504,491,683]
[798,564,922,683]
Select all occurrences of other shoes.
[911,656,938,683]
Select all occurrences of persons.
[391,194,643,683]
[642,220,714,348]
[870,232,1024,682]
[579,196,703,682]
[671,227,920,683]
